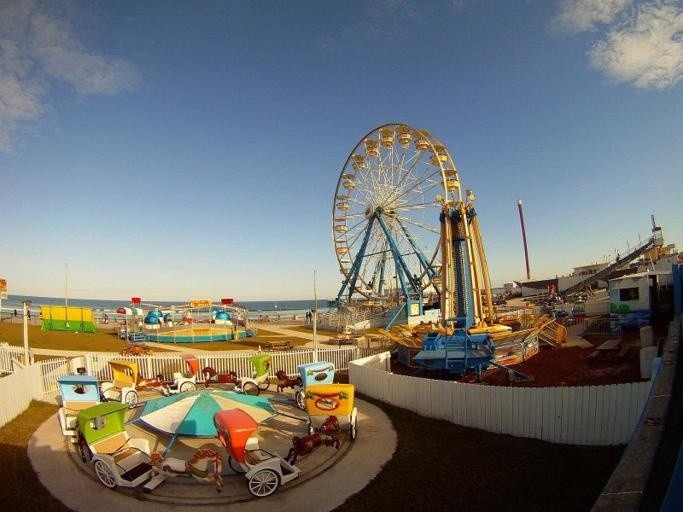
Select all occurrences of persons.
[309,311,312,322]
[305,312,308,325]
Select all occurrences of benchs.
[167,372,192,393]
[246,437,296,478]
[58,400,101,436]
[262,341,294,353]
[89,431,153,484]
[100,380,135,403]
